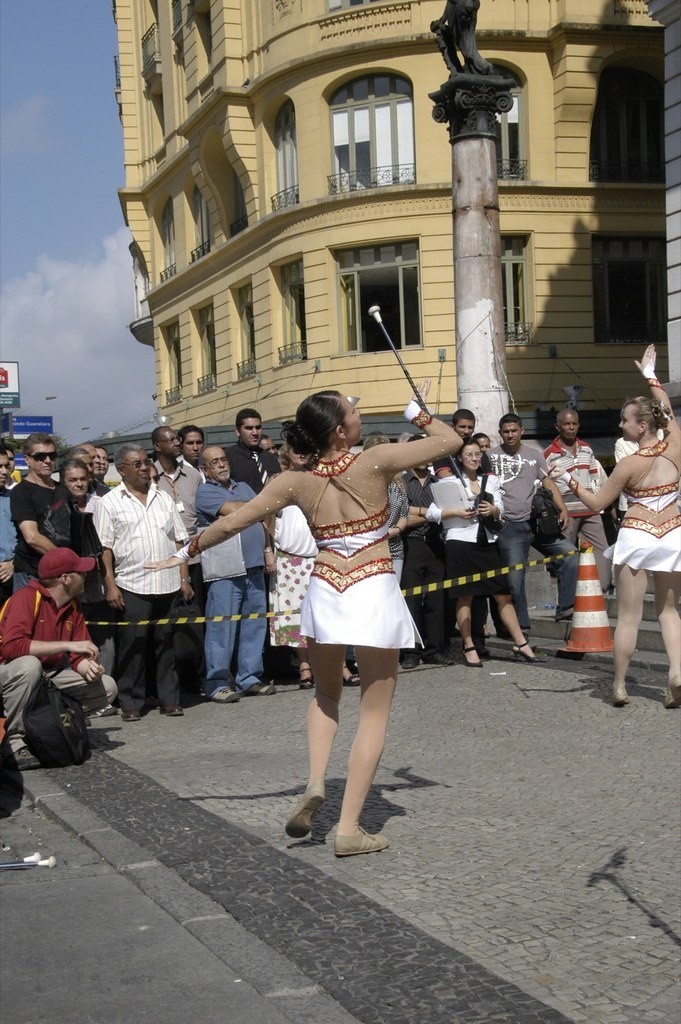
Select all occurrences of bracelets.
[419,507,421,515]
[182,576,192,583]
[264,549,272,553]
[392,526,402,535]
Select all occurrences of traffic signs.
[0,412,53,439]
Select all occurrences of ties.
[251,451,269,484]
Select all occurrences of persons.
[142,379,463,856]
[539,344,681,708]
[0,406,666,770]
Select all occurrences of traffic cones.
[559,540,614,653]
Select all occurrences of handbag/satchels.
[165,593,204,660]
[474,476,502,534]
[532,488,560,536]
[23,673,89,767]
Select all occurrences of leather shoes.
[160,705,183,716]
[122,710,140,721]
[402,654,419,668]
[422,652,454,665]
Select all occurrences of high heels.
[462,644,483,667]
[512,642,546,662]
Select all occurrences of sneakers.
[209,686,239,703]
[334,827,389,855]
[239,683,276,695]
[285,780,325,838]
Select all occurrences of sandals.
[343,666,361,686]
[300,668,313,689]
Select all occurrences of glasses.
[120,460,151,468]
[154,435,182,445]
[27,452,58,462]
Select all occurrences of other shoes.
[613,687,629,705]
[665,674,681,709]
[555,606,574,622]
[520,627,532,644]
[91,704,117,717]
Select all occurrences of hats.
[38,548,96,579]
